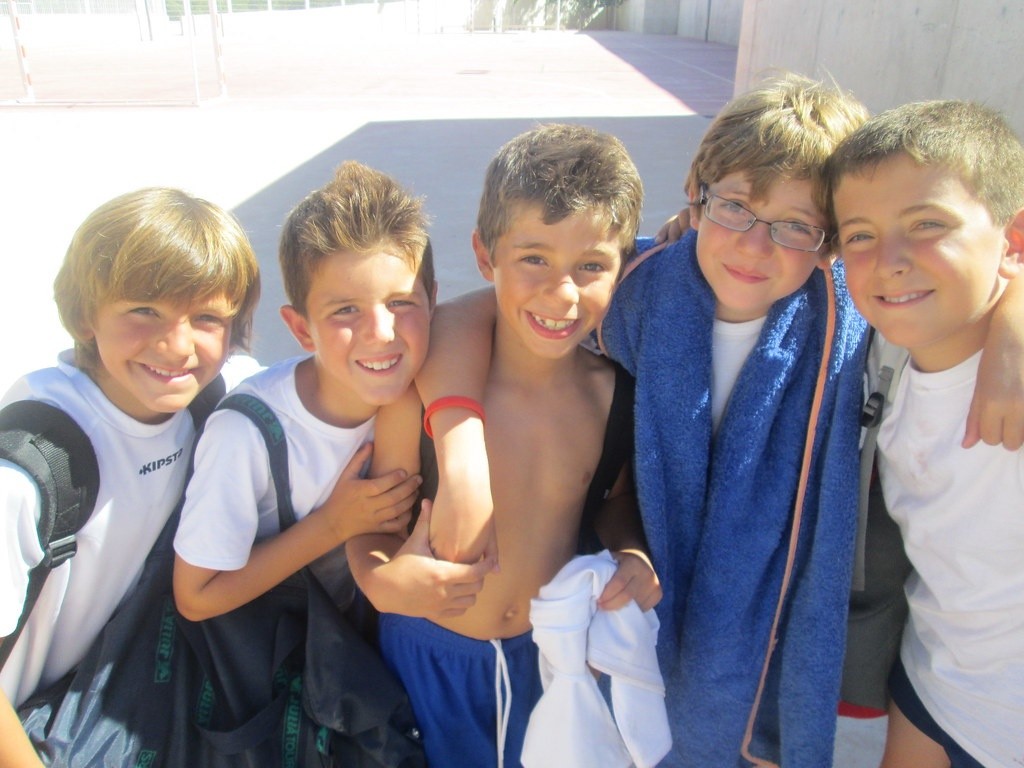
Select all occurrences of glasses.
[699,183,833,252]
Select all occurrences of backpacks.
[835,335,917,718]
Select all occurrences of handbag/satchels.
[17,394,430,768]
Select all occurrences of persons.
[414,68,1024,768]
[0,159,440,768]
[346,122,664,767]
[654,97,1024,768]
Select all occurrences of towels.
[519,547,675,768]
[591,230,871,768]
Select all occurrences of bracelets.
[424,396,485,439]
